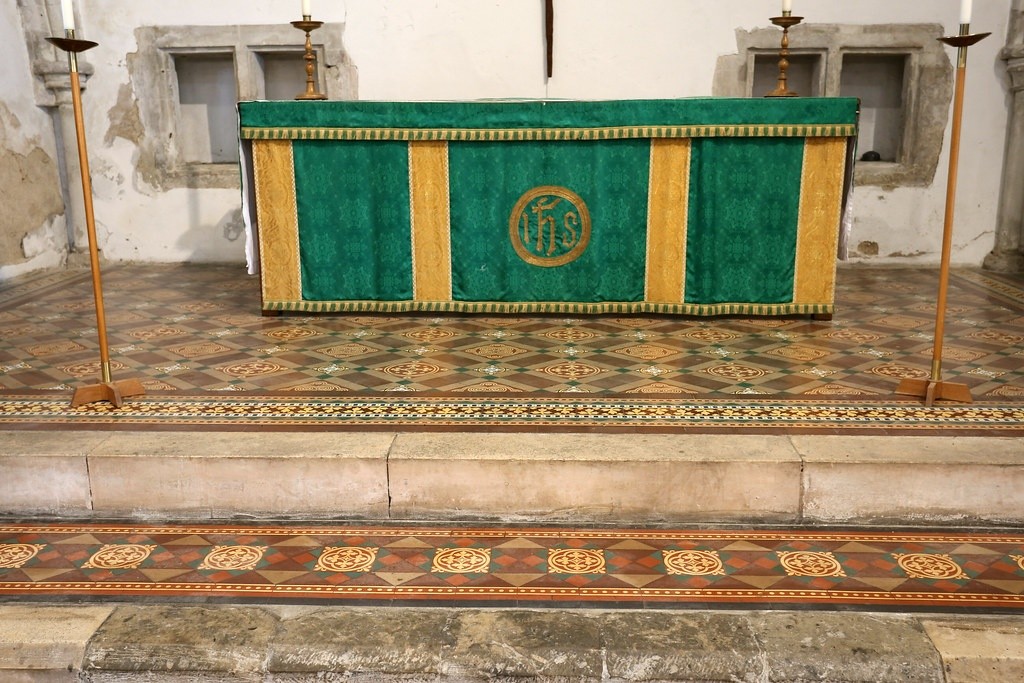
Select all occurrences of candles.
[782,0,791,11]
[61,0,75,29]
[302,0,311,15]
[960,0,972,24]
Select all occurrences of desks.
[235,98,860,320]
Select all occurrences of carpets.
[0,262,1024,436]
[0,514,1024,613]
